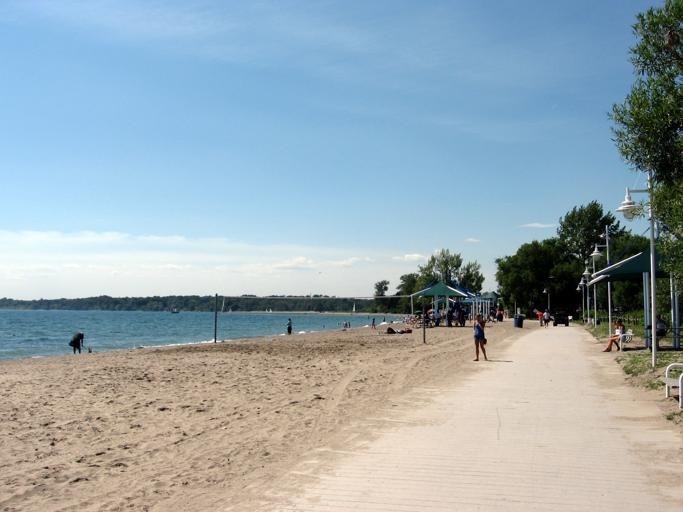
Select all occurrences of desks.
[652,327,683,347]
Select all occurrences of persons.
[543,309,550,328]
[489,308,507,323]
[387,309,466,334]
[343,320,350,328]
[473,313,488,361]
[372,318,376,329]
[610,320,620,351]
[656,315,669,337]
[602,319,625,352]
[287,318,292,335]
[68,332,84,354]
[536,311,543,326]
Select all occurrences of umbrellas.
[595,250,670,347]
[408,282,495,320]
[586,275,648,349]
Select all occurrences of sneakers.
[473,358,488,361]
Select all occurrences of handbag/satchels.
[483,339,487,344]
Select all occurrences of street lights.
[571,149,659,366]
[542,286,551,315]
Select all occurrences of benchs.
[644,336,683,347]
[612,329,636,351]
[406,316,433,328]
[666,363,683,409]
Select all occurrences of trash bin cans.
[514,314,523,328]
